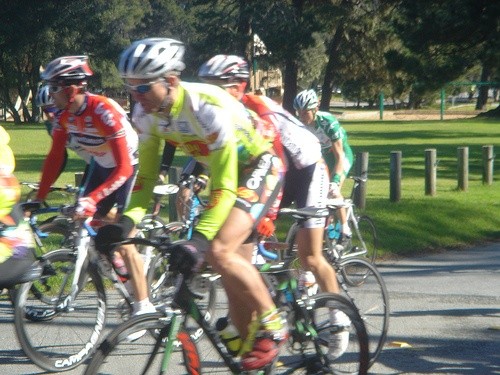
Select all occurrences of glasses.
[122,77,167,93]
[47,85,71,93]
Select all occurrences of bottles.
[216,316,246,357]
[110,250,128,281]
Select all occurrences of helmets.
[117,37,186,79]
[196,54,251,80]
[35,86,56,107]
[293,88,320,111]
[40,55,93,83]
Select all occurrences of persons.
[0,37,354,371]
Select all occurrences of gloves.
[329,181,342,200]
[73,196,97,221]
[95,214,136,254]
[168,230,210,278]
[256,215,276,238]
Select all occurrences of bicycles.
[259,191,378,287]
[184,173,390,375]
[11,169,221,374]
[85,226,368,375]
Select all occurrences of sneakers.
[334,229,353,251]
[327,312,352,360]
[298,271,318,310]
[126,303,156,342]
[240,322,290,370]
